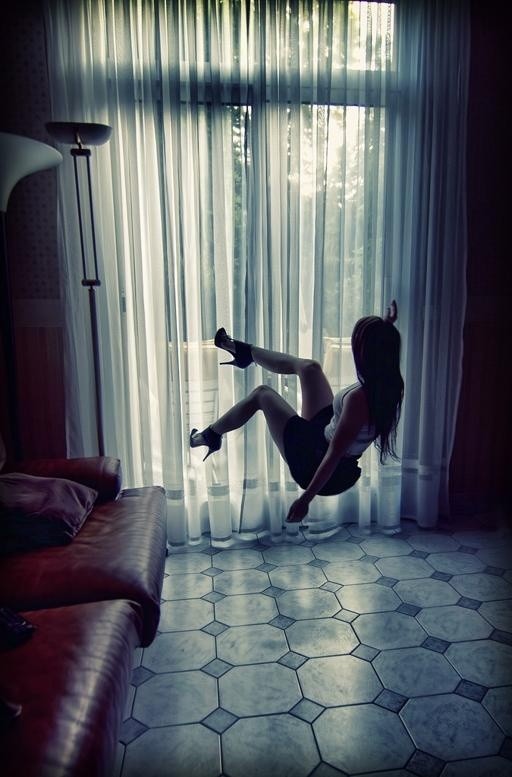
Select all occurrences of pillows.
[0,470,98,546]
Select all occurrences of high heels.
[215,328,255,368]
[190,425,222,461]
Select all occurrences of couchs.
[2,599,136,775]
[1,457,170,642]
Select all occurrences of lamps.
[45,121,116,460]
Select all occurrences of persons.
[190,299,404,523]
